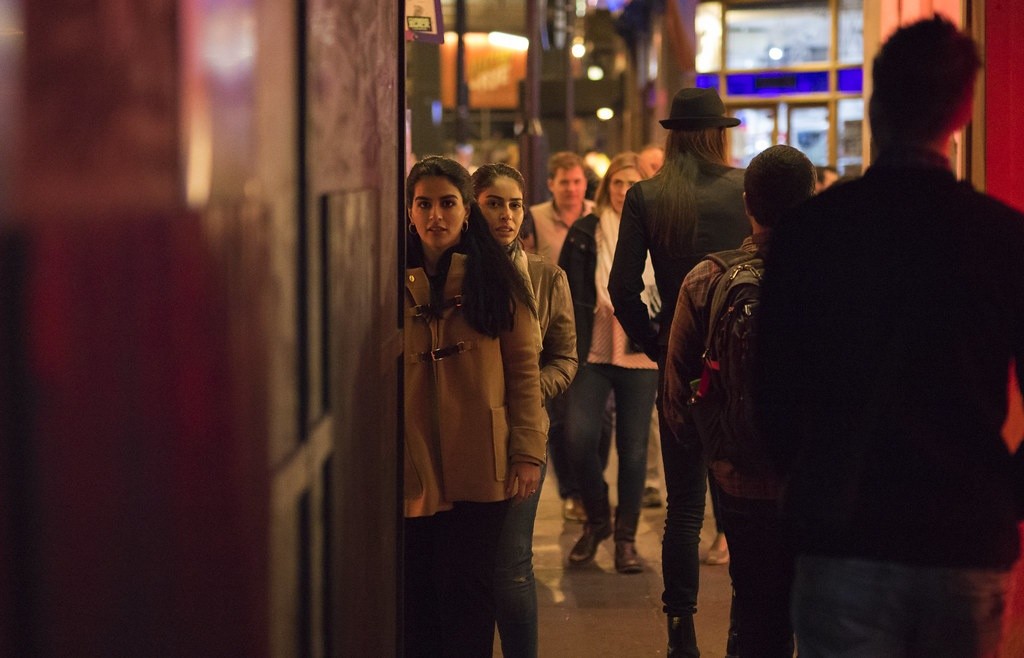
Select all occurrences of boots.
[570,499,612,563]
[615,507,643,573]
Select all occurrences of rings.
[531,490,536,494]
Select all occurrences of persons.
[402,155,548,658]
[704,467,729,565]
[663,145,818,658]
[606,89,750,657]
[469,163,580,658]
[548,151,662,573]
[641,148,665,511]
[760,13,1024,657]
[521,152,595,264]
[813,166,838,194]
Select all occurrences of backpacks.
[689,249,787,507]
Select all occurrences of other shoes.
[640,487,662,505]
[706,533,729,565]
[665,608,701,658]
[565,501,585,522]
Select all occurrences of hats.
[659,86,742,129]
